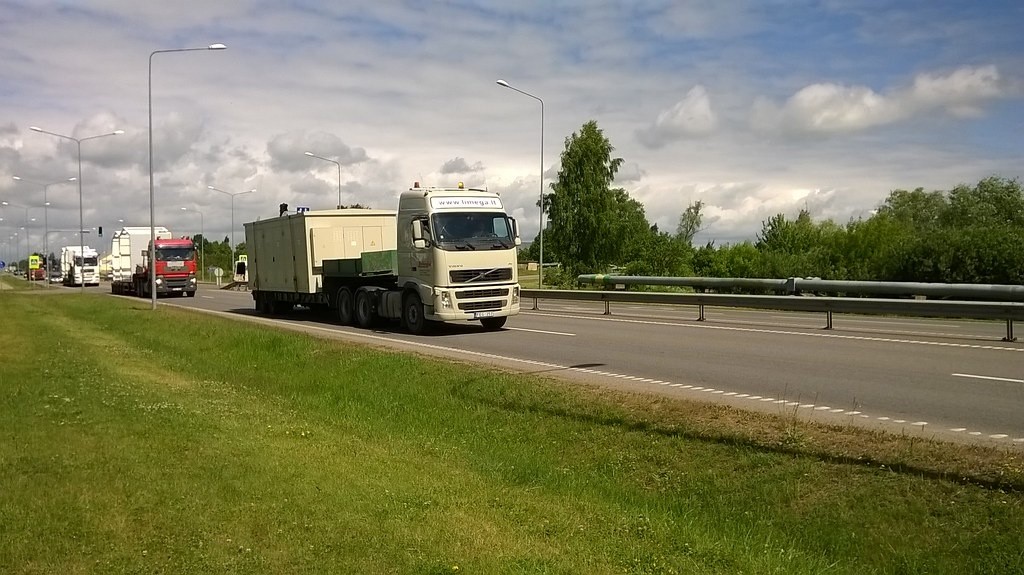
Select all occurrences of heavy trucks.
[241,183,521,334]
[111,236,198,299]
[62,251,101,287]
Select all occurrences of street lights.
[304,151,341,210]
[496,79,545,290]
[181,207,204,282]
[208,184,256,278]
[0,177,77,289]
[147,42,226,309]
[28,126,126,286]
